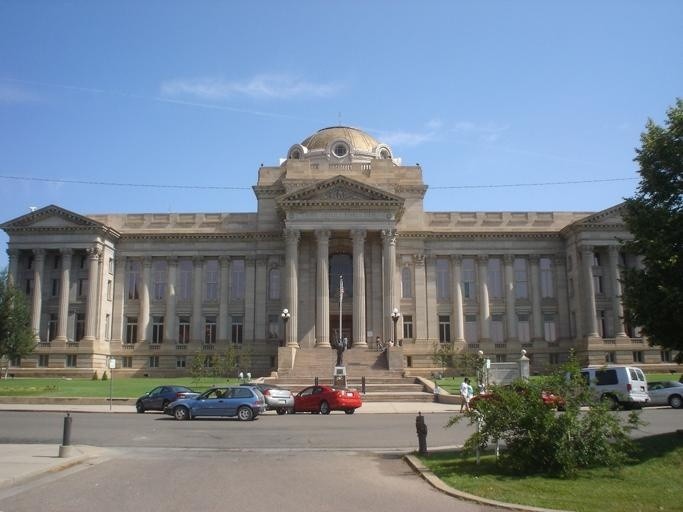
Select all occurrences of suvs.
[240,383,295,415]
[168,386,267,421]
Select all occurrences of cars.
[288,385,362,414]
[135,386,201,414]
[641,381,683,409]
[469,385,557,410]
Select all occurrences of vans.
[564,366,649,411]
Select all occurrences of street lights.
[108,356,116,411]
[281,308,290,346]
[391,307,400,347]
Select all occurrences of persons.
[216,391,224,399]
[376,337,394,352]
[459,376,486,414]
[343,335,348,351]
